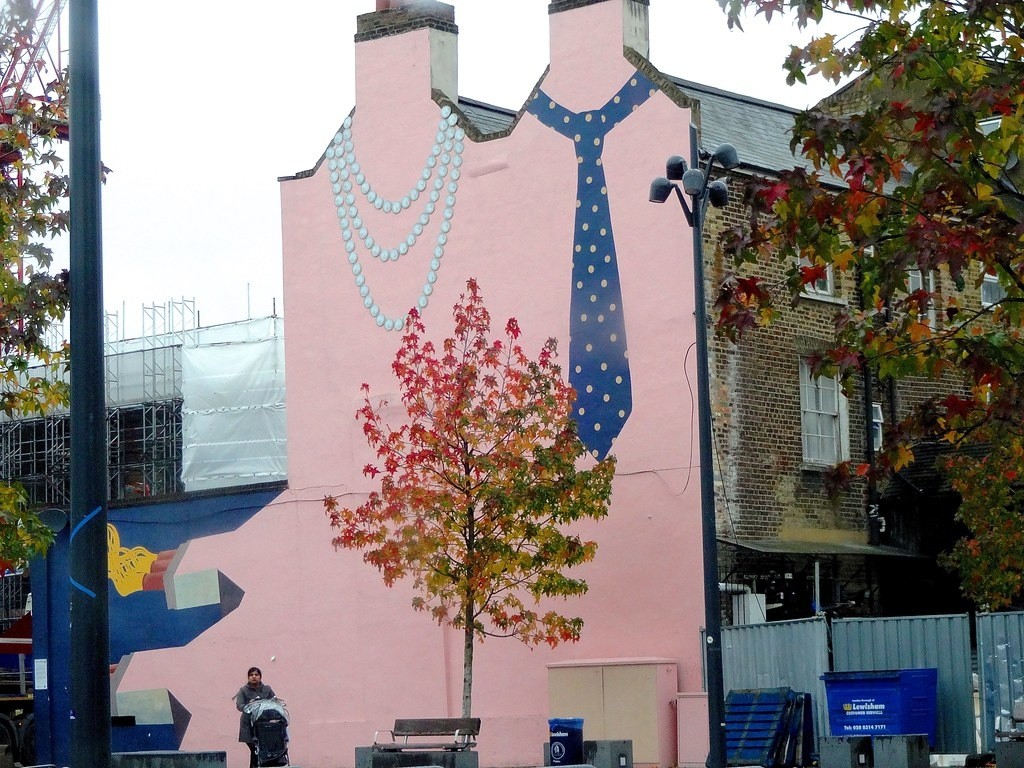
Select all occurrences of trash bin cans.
[820,668,940,754]
[548,717,584,766]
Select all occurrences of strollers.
[248,697,289,768]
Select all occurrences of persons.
[237,667,276,768]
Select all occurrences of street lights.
[652,141,744,760]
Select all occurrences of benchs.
[371,717,481,750]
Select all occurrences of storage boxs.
[819,666,939,749]
